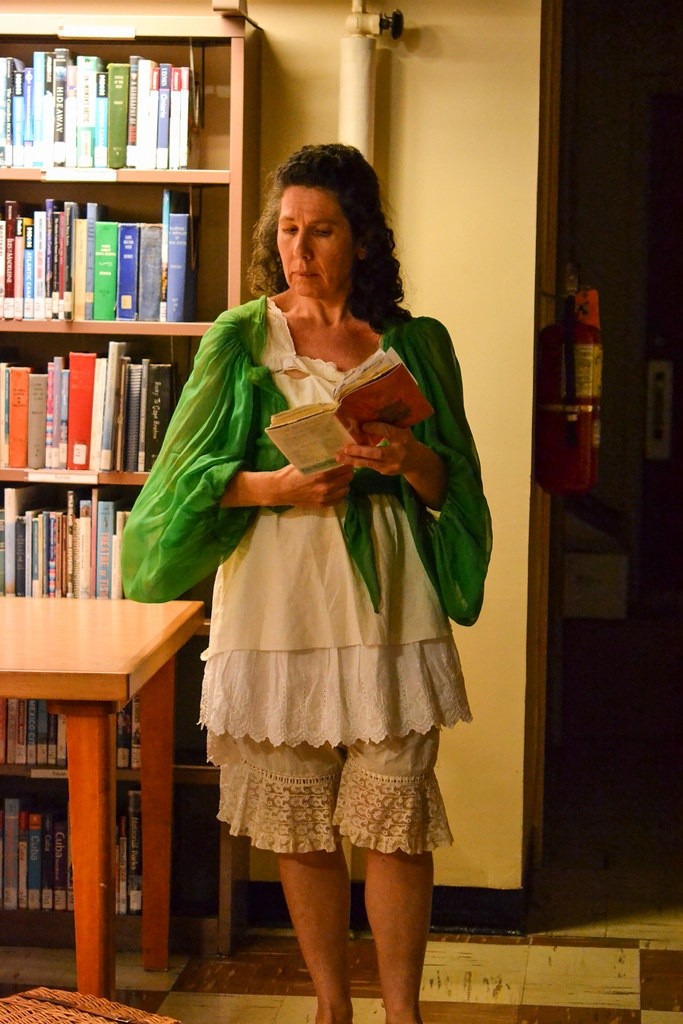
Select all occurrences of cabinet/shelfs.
[0,12,252,959]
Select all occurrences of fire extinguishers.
[534,270,604,496]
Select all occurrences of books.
[0,486,131,600]
[0,188,198,322]
[0,340,177,472]
[0,789,142,915]
[264,346,435,479]
[6,694,142,769]
[0,48,199,170]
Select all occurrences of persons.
[121,143,493,1024]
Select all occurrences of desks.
[1,594,204,1004]
